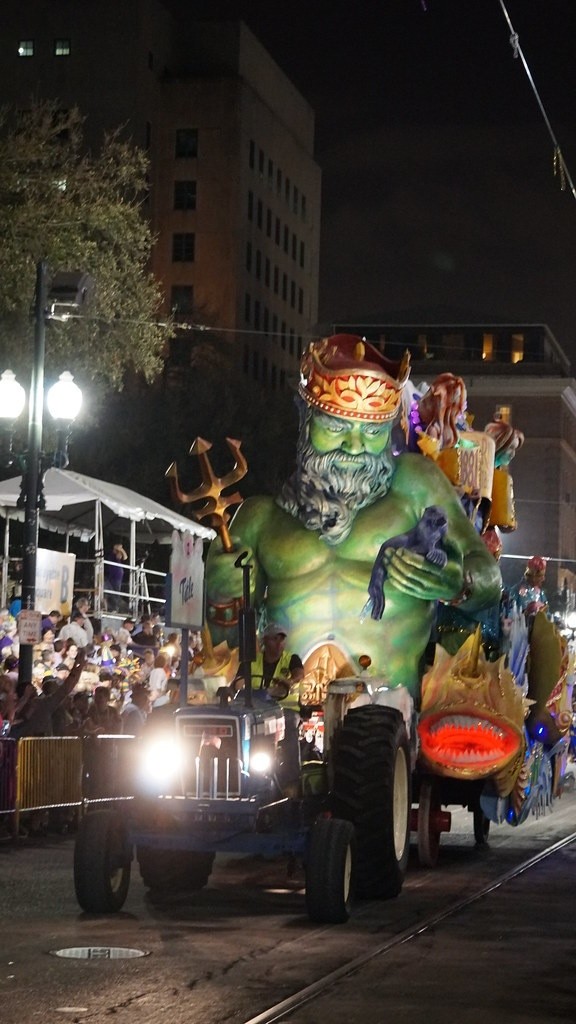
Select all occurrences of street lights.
[15,258,88,700]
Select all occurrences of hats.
[5,655,19,671]
[73,692,91,702]
[528,555,547,570]
[264,623,288,642]
[130,685,150,698]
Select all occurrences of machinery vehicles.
[71,550,414,924]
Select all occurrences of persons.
[109,544,127,595]
[509,556,558,623]
[229,624,303,783]
[0,597,203,838]
[206,334,503,706]
